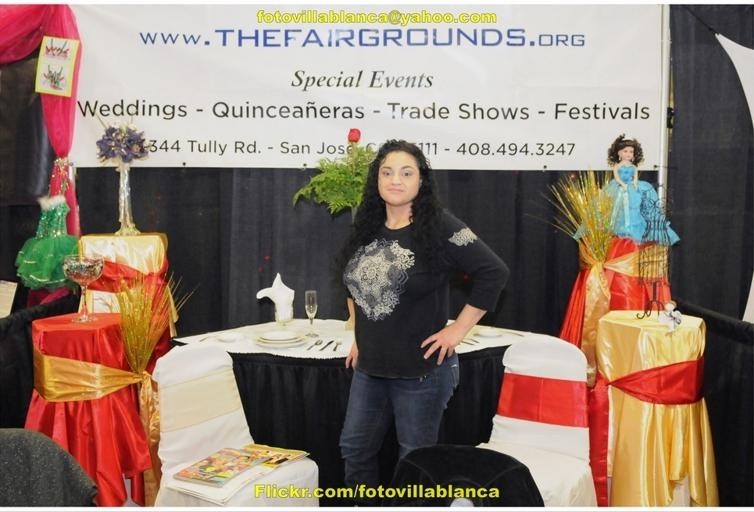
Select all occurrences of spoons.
[306,335,343,352]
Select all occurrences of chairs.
[156,342,318,507]
[0,429,71,506]
[385,446,545,507]
[476,335,590,507]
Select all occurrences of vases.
[114,164,141,235]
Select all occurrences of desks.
[578,236,704,507]
[32,232,168,507]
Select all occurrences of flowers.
[88,106,151,163]
[289,128,381,214]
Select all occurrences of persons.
[573,133,681,247]
[338,138,512,487]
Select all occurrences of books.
[165,441,312,505]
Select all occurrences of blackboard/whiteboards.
[64,4,669,172]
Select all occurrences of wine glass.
[302,289,319,334]
[63,254,103,323]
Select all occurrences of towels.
[256,272,295,321]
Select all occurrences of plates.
[477,325,504,339]
[253,327,306,351]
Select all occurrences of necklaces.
[385,220,411,229]
